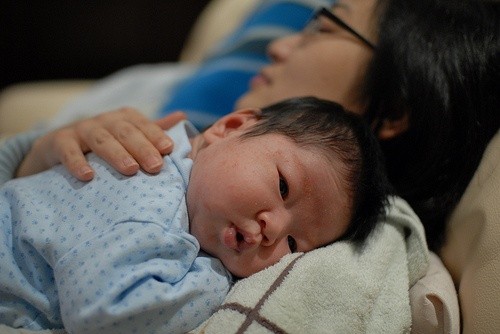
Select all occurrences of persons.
[0,0,500,334]
[1,95,391,334]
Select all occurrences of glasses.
[312,6,373,53]
